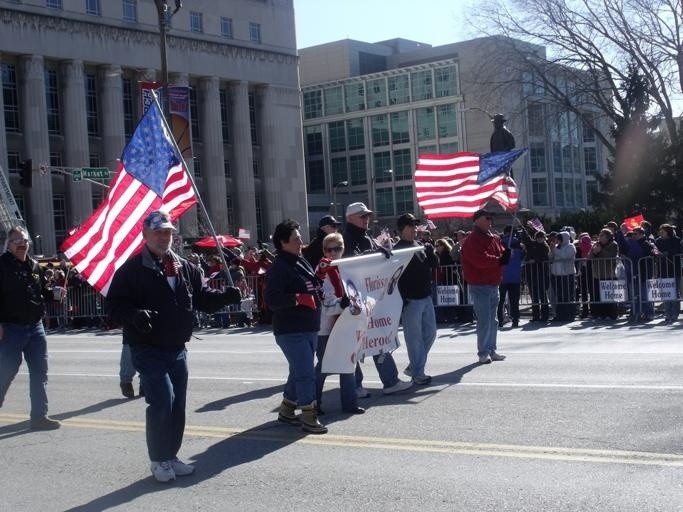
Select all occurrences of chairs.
[138,384,147,396]
[31,417,60,431]
[404,366,432,384]
[120,381,134,399]
[171,458,194,476]
[479,356,491,363]
[356,387,372,398]
[151,457,176,483]
[383,380,414,394]
[432,306,680,328]
[490,353,505,361]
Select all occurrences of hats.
[30,255,75,270]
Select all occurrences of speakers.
[278,398,301,425]
[300,400,329,433]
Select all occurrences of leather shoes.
[377,246,393,258]
[296,294,318,309]
[135,308,159,332]
[498,247,512,266]
[222,286,243,308]
[425,242,434,257]
[340,293,350,310]
[319,257,333,275]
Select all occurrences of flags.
[60,101,196,298]
[410,148,526,218]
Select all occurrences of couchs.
[17,158,32,188]
[71,169,82,183]
[81,166,110,179]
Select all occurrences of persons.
[188,247,276,326]
[265,219,331,433]
[381,235,400,249]
[393,213,440,385]
[314,233,365,413]
[41,260,119,330]
[120,328,146,396]
[461,208,511,363]
[106,209,241,482]
[419,229,475,321]
[490,114,517,184]
[304,216,344,267]
[497,223,683,328]
[343,203,414,397]
[1,226,67,428]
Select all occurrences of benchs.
[60,101,196,298]
[410,148,526,218]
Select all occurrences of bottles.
[534,231,545,240]
[144,210,178,233]
[345,201,373,216]
[473,209,495,222]
[397,213,420,232]
[319,214,341,227]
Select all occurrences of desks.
[368,167,393,222]
[456,106,495,123]
[329,202,344,223]
[329,180,347,226]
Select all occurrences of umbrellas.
[343,405,365,414]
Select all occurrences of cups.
[8,238,29,246]
[324,246,344,253]
[358,213,369,219]
